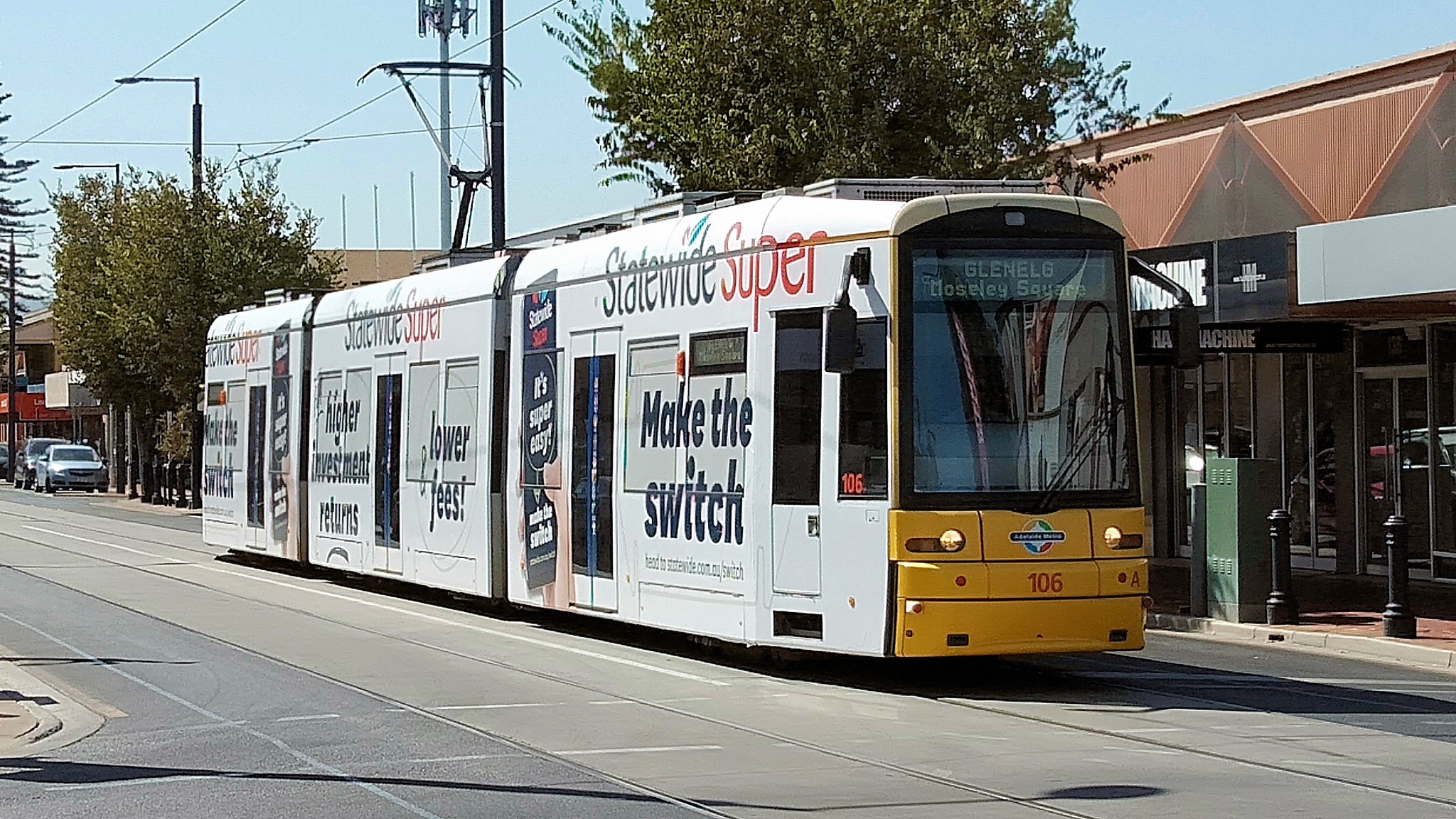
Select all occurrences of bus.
[201,63,1196,677]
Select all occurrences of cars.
[1288,426,1456,564]
[0,441,8,481]
[34,444,110,494]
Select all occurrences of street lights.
[56,163,122,227]
[115,77,204,510]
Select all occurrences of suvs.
[12,435,71,490]
